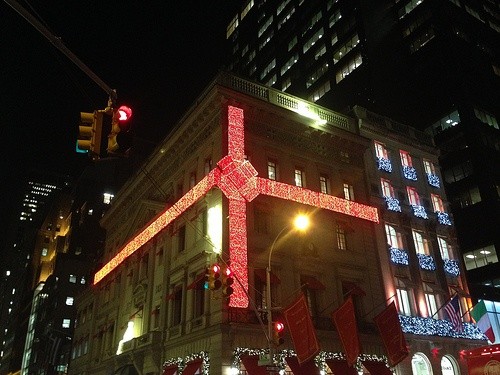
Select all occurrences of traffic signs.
[265,364,283,372]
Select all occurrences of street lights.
[266,211,313,375]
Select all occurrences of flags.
[331,298,363,367]
[283,294,320,365]
[373,300,409,365]
[444,293,463,334]
[468,301,495,344]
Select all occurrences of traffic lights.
[204,264,222,291]
[75,110,106,158]
[274,321,285,346]
[107,103,138,161]
[221,266,233,296]
